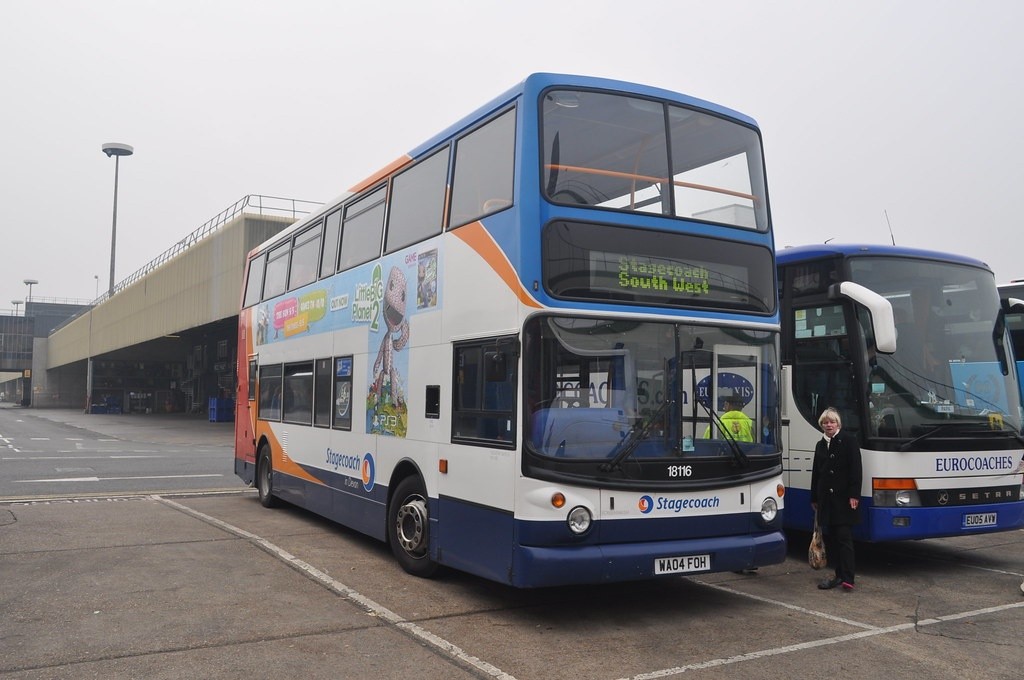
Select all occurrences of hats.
[720,389,744,407]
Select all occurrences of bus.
[232,71,783,587]
[557,241,1024,545]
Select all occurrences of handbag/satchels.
[808,509,828,570]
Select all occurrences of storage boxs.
[92,406,105,414]
[208,396,233,422]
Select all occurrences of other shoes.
[835,578,847,585]
[817,575,838,589]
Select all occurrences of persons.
[810,408,862,590]
[705,396,758,573]
[869,307,924,372]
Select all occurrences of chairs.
[481,381,528,443]
[448,199,511,227]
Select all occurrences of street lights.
[24,278,39,302]
[101,142,133,298]
[12,299,23,317]
